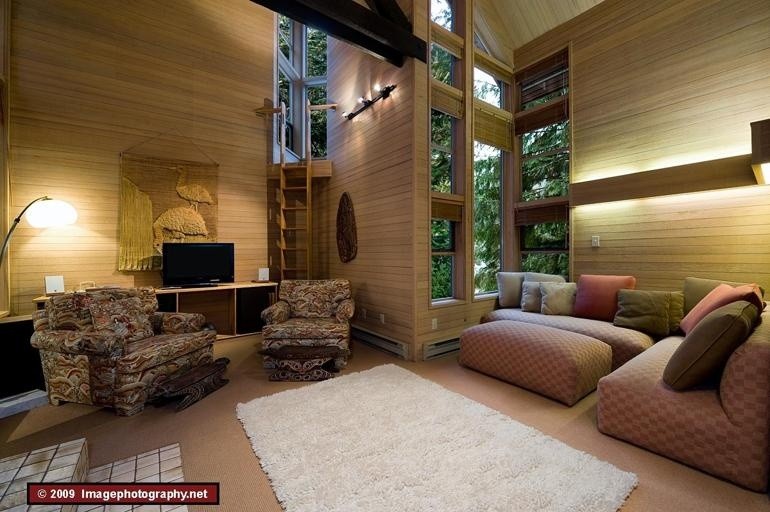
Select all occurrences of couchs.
[479,248,654,353]
[596,300,770,500]
[31,287,228,420]
[259,280,353,382]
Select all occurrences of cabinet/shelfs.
[157,280,277,341]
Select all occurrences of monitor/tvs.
[162,241,236,288]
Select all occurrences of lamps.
[1,195,78,276]
[341,112,356,119]
[357,98,374,106]
[372,84,390,96]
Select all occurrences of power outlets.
[431,319,439,328]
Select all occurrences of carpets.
[239,363,635,512]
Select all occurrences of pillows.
[660,299,756,396]
[682,275,734,308]
[681,283,745,336]
[293,284,331,316]
[672,292,685,337]
[87,298,153,343]
[613,288,671,337]
[578,275,636,319]
[525,271,565,283]
[522,281,553,317]
[498,270,524,309]
[540,281,577,315]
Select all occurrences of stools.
[460,319,614,409]
